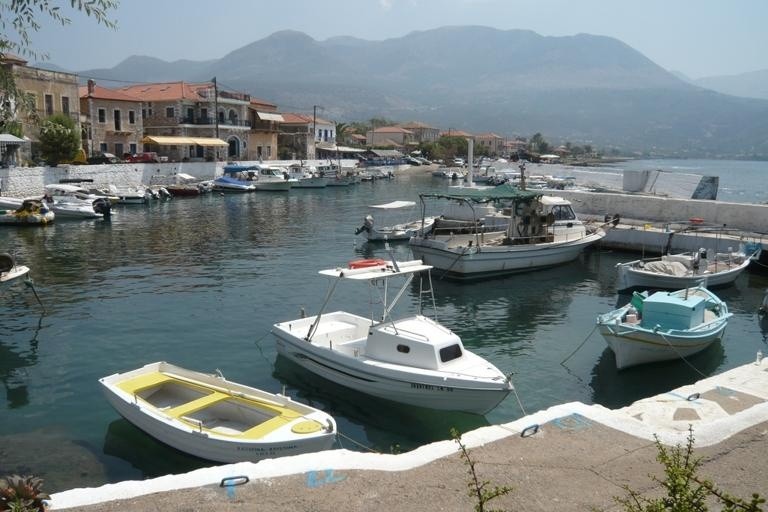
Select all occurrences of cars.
[414,157,432,165]
[88,152,118,164]
[452,158,463,168]
[403,157,422,166]
[125,152,157,163]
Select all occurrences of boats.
[354,200,443,243]
[614,246,750,292]
[96,361,337,464]
[431,168,596,192]
[409,182,606,280]
[595,285,728,370]
[270,259,514,416]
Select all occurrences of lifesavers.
[689,217,705,221]
[350,259,384,269]
